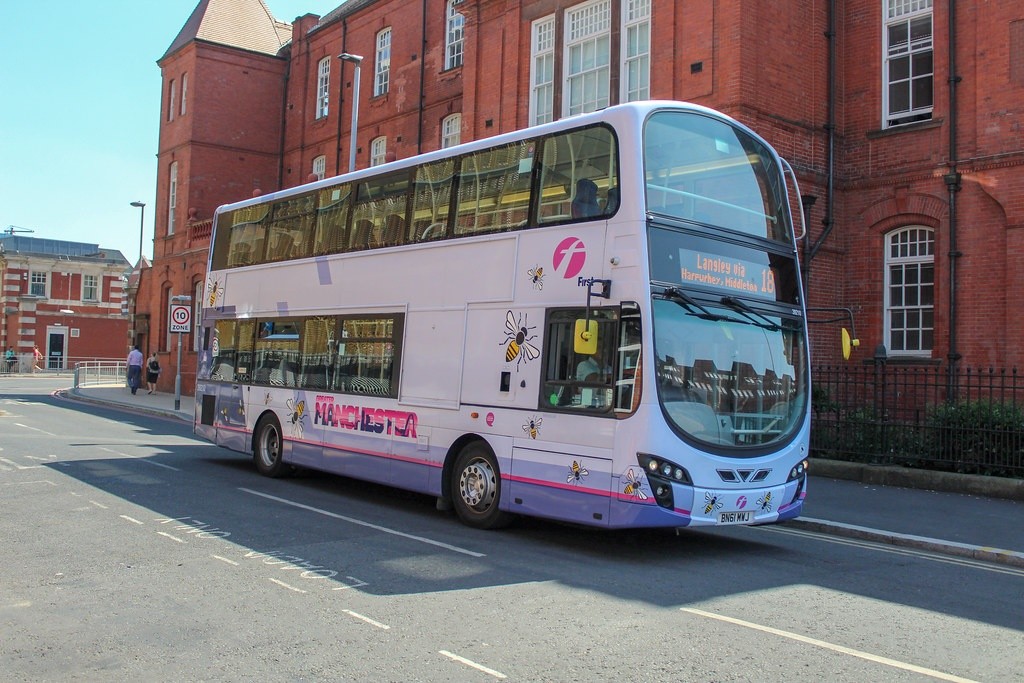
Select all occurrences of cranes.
[3,224,35,235]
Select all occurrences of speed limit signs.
[172,306,190,325]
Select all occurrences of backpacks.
[149,361,160,370]
[38,351,43,359]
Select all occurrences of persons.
[33,345,42,373]
[6,346,15,372]
[125,345,143,395]
[577,336,613,405]
[146,351,160,395]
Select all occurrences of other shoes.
[151,393,156,395]
[148,390,152,394]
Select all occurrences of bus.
[192,101,861,532]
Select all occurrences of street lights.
[337,52,365,174]
[129,200,146,270]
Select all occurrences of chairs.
[570,178,602,219]
[603,188,618,215]
[230,215,434,266]
[210,356,393,395]
